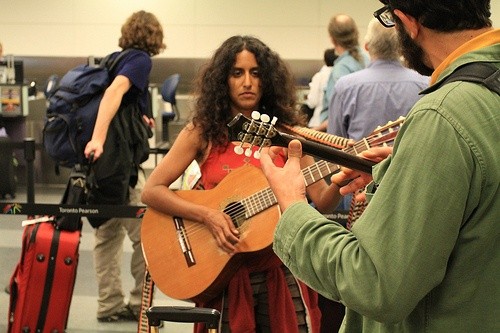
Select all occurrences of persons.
[259,0,500,332]
[328,12,437,234]
[311,13,375,133]
[310,49,341,117]
[141,34,356,333]
[79,10,166,327]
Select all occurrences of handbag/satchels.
[55,163,86,233]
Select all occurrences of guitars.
[139,115,407,304]
[227,110,378,174]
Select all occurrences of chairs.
[161,74,180,142]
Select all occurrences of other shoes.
[128,304,163,327]
[96,307,134,322]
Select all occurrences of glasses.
[373,4,398,28]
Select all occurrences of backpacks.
[44,49,140,175]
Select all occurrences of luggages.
[7,151,94,332]
